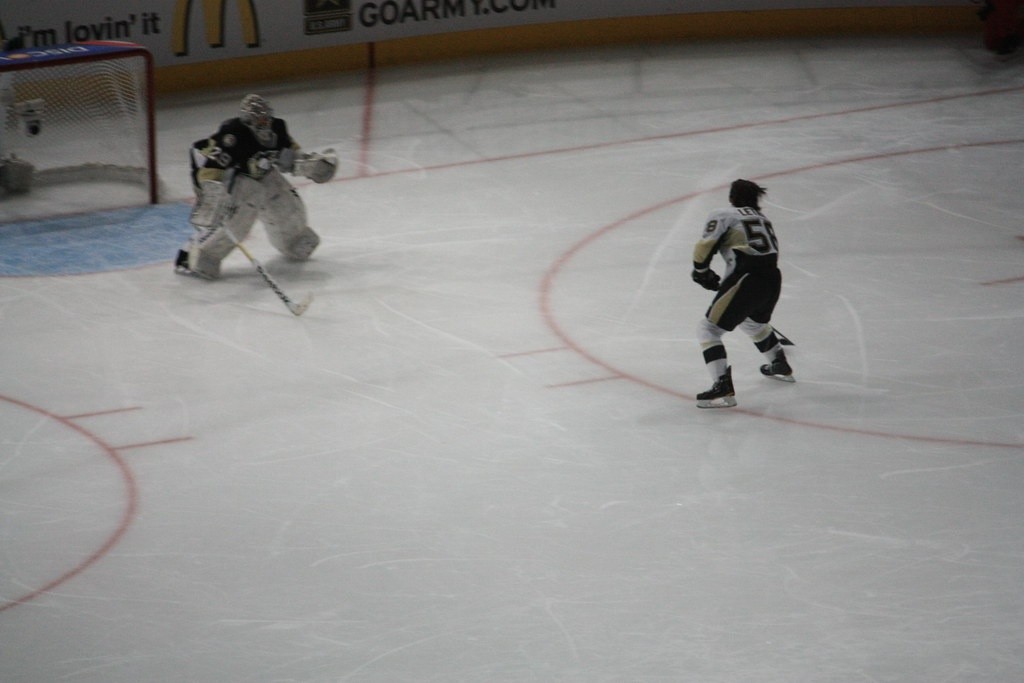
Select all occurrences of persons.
[692,179,794,401]
[189,95,339,280]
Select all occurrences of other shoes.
[186,175,265,280]
[259,169,320,260]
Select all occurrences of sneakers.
[760,348,793,375]
[696,365,736,400]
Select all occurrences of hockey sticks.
[719,288,794,346]
[221,226,313,316]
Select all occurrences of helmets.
[240,94,271,127]
